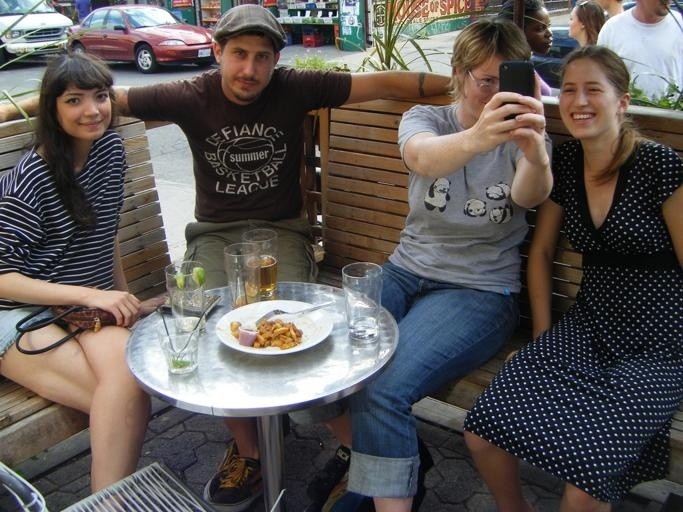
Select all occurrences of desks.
[125,277,399,512]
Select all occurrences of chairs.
[0,461,212,512]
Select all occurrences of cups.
[341,261,385,345]
[156,257,210,378]
[221,229,278,308]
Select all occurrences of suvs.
[0,0,76,69]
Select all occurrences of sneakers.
[307,441,356,499]
[204,439,263,512]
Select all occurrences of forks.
[255,297,337,327]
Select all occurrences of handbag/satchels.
[13,301,117,355]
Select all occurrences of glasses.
[579,0,590,9]
[466,69,499,97]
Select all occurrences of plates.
[215,299,334,357]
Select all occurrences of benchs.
[0,110,177,471]
[313,89,682,507]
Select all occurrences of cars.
[65,4,216,74]
[530,0,682,87]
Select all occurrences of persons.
[462,45,683,512]
[347,16,554,512]
[0,52,168,496]
[0,4,457,511]
[497,0,683,106]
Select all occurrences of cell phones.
[498,60,535,119]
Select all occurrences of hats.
[212,3,290,53]
